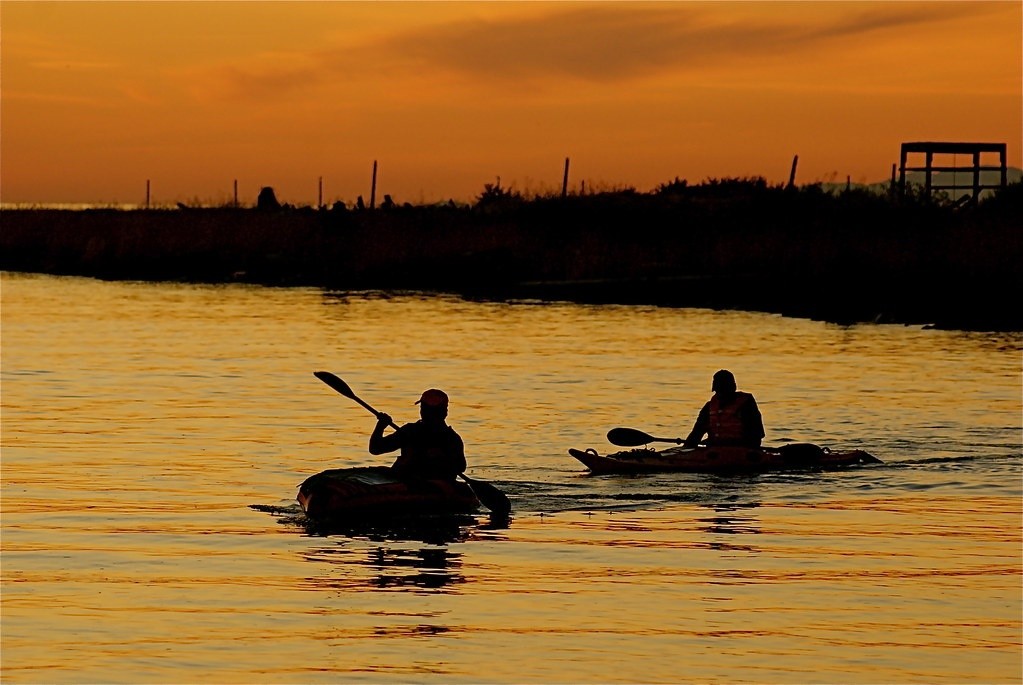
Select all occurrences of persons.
[369,389,466,480]
[682,370,765,448]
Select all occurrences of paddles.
[606,426,822,454]
[314,368,514,516]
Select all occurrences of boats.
[566,445,866,474]
[294,465,481,523]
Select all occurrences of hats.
[712,370,735,392]
[415,389,448,408]
